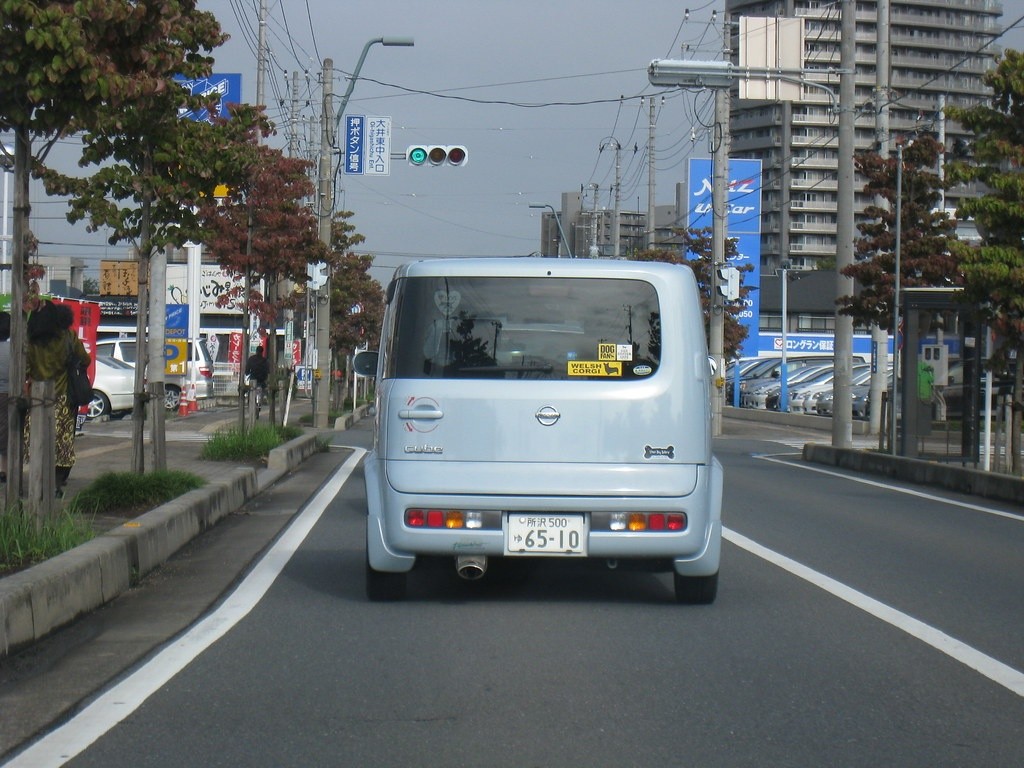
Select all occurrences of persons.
[0,311,11,483]
[245,346,269,421]
[25,299,91,498]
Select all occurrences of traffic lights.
[716,268,741,303]
[405,145,468,168]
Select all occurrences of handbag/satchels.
[244,374,251,390]
[66,328,94,406]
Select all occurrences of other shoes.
[56,487,63,499]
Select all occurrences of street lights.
[647,54,852,454]
[312,35,417,431]
[528,201,573,260]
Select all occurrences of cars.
[72,353,145,420]
[725,355,1014,421]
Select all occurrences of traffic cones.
[176,386,191,417]
[187,383,200,412]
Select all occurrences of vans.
[353,258,724,607]
[95,336,216,412]
